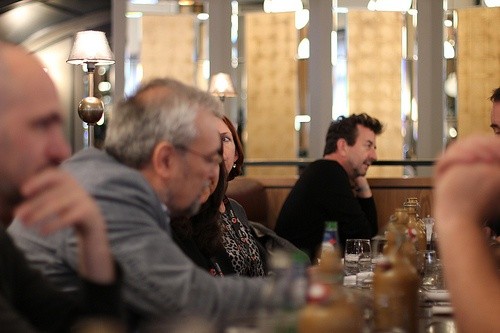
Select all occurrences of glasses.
[177,145,222,165]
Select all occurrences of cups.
[416,249,443,289]
[343,239,373,294]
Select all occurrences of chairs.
[223,176,270,232]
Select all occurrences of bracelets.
[356,184,368,192]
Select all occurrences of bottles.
[297,220,365,333]
[372,198,427,333]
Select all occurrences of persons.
[486,87,500,243]
[171,111,269,278]
[0,42,124,333]
[7,78,309,325]
[433,137,500,333]
[274,114,382,264]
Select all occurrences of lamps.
[67,30,118,97]
[210,73,237,104]
